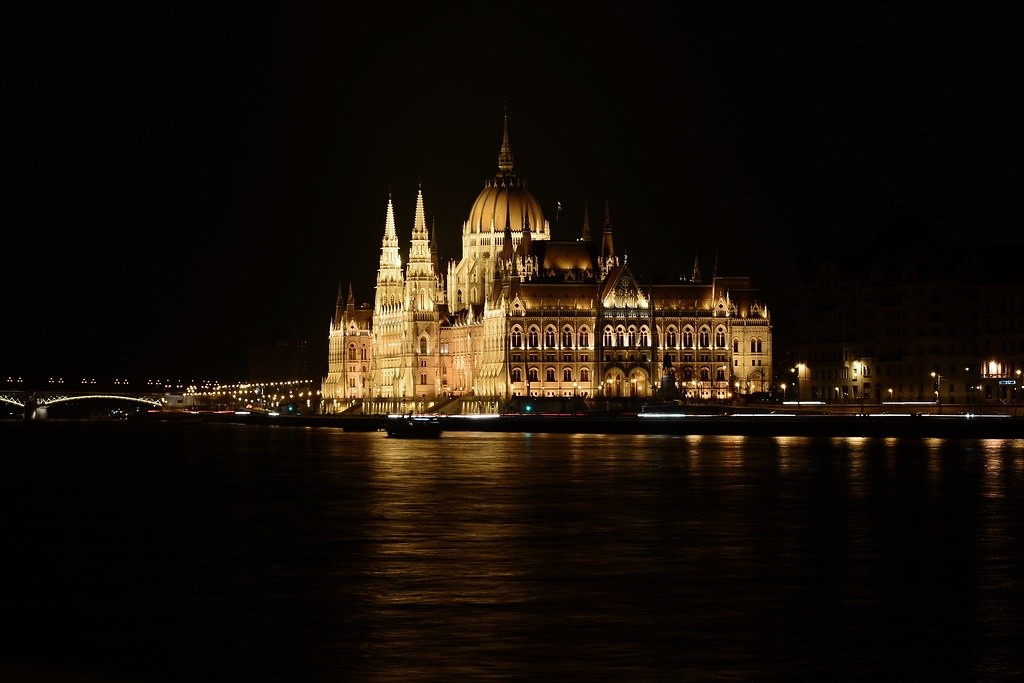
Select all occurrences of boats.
[160,412,202,424]
[384,413,442,438]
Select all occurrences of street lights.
[123,379,129,393]
[854,361,863,413]
[147,380,153,393]
[791,367,799,405]
[82,378,87,391]
[58,378,64,392]
[931,372,941,415]
[114,379,120,392]
[17,377,22,391]
[156,380,161,394]
[90,379,96,392]
[6,377,12,391]
[165,380,171,393]
[49,378,54,391]
[177,380,182,392]
